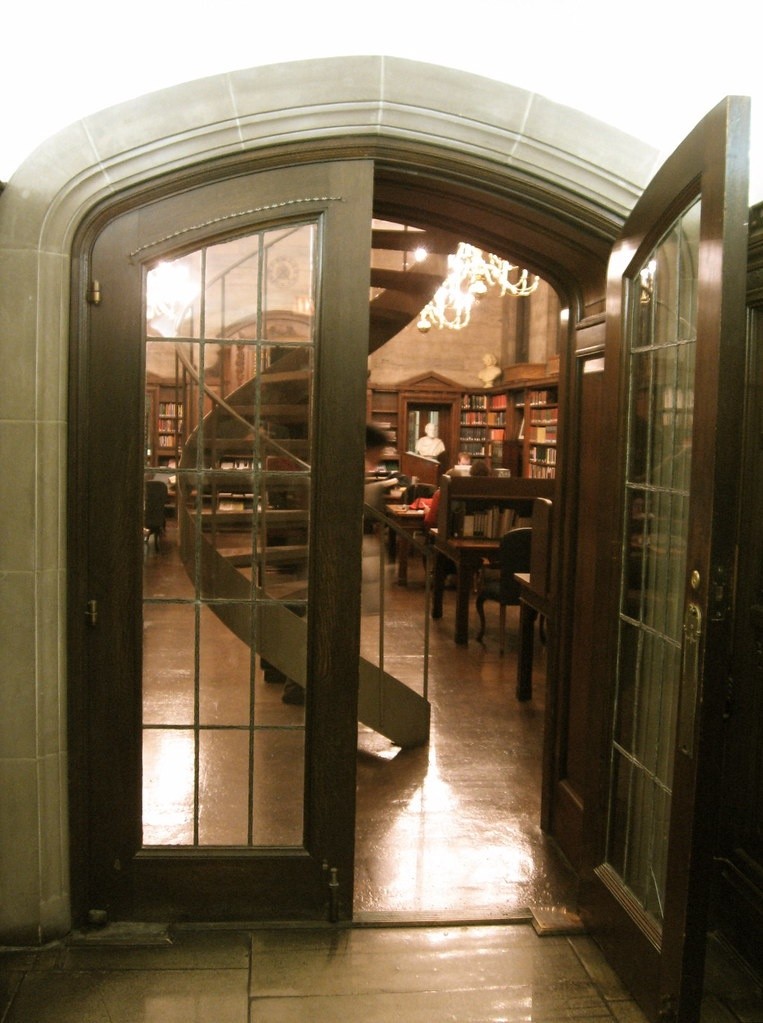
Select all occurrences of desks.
[387,504,429,586]
[430,474,558,644]
[514,498,555,703]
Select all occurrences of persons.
[652,421,692,515]
[424,452,471,587]
[415,423,446,457]
[478,353,501,382]
[469,461,490,476]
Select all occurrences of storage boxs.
[504,363,548,383]
[545,355,560,374]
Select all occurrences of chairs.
[144,480,167,550]
[476,529,547,654]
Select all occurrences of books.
[159,402,182,447]
[460,394,507,457]
[463,505,531,539]
[515,391,558,479]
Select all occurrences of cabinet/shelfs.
[146,371,265,510]
[366,370,560,480]
